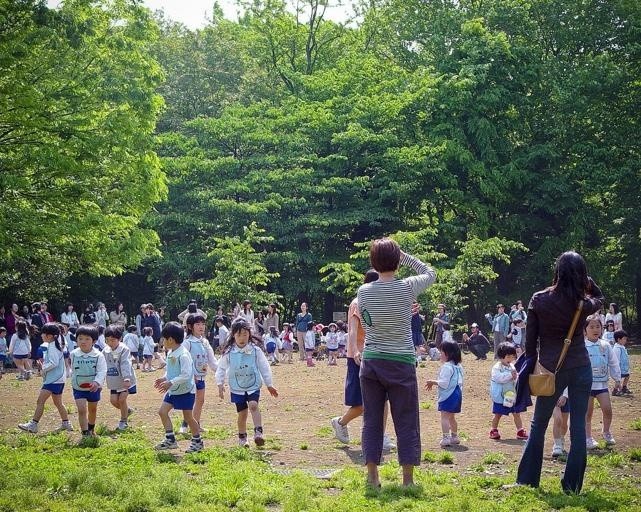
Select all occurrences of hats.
[327,323,338,330]
[436,304,446,309]
[469,323,478,328]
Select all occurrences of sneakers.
[551,437,564,457]
[153,436,177,450]
[586,437,599,450]
[622,387,633,394]
[115,421,127,432]
[15,373,24,380]
[501,482,534,490]
[481,355,487,360]
[157,362,167,369]
[440,434,451,446]
[611,389,624,396]
[270,359,280,366]
[185,439,204,454]
[17,420,37,434]
[489,428,501,439]
[128,406,135,418]
[238,437,250,449]
[23,370,31,381]
[365,476,382,491]
[199,426,209,432]
[253,433,266,447]
[54,420,74,433]
[81,428,97,443]
[602,429,616,445]
[450,435,461,445]
[141,368,148,372]
[516,427,529,440]
[137,364,140,369]
[475,355,481,360]
[179,424,188,434]
[327,359,336,366]
[382,433,396,451]
[148,368,156,372]
[331,417,350,445]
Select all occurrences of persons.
[583,302,633,450]
[331,268,395,450]
[412,301,527,361]
[359,237,437,488]
[490,343,529,440]
[503,252,606,496]
[424,340,465,447]
[19,315,278,454]
[552,387,569,463]
[1,300,349,381]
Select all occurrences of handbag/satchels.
[525,361,556,397]
